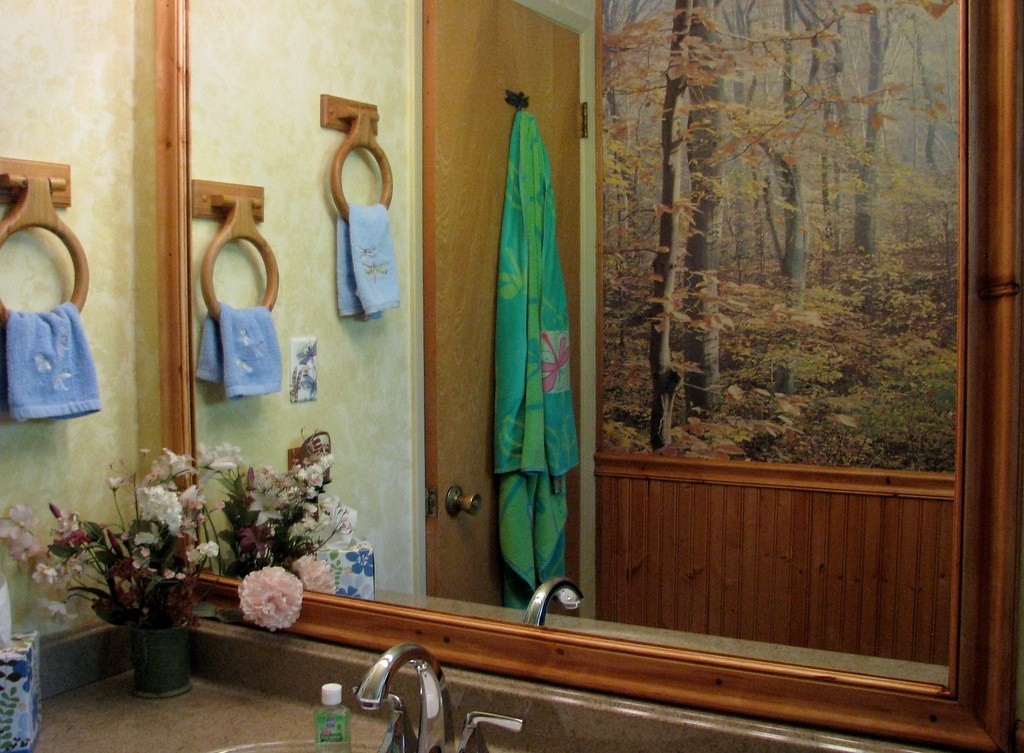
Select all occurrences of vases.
[132,622,195,700]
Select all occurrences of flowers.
[209,452,355,632]
[1,445,221,628]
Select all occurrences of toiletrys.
[312,682,349,748]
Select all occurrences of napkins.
[313,503,379,600]
[0,579,45,753]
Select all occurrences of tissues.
[0,571,47,752]
[309,493,378,600]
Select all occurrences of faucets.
[351,640,457,753]
[519,574,585,633]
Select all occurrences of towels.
[194,301,289,400]
[491,107,580,615]
[332,201,404,321]
[0,299,103,427]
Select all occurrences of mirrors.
[149,0,1024,753]
